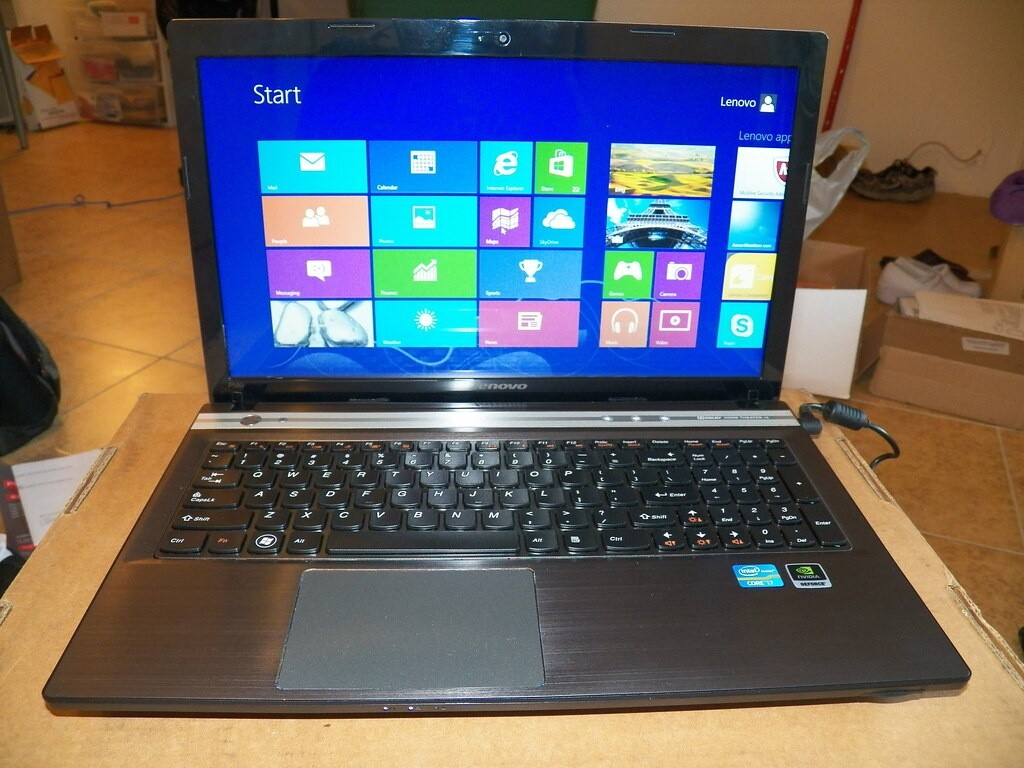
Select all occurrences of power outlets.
[967,142,993,168]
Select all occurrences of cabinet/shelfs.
[70,34,164,127]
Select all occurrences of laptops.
[39,19,972,710]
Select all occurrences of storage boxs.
[983,225,1024,302]
[100,11,149,37]
[870,302,1024,432]
[0,387,1024,768]
[796,240,886,383]
[6,24,80,132]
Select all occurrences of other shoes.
[851,159,924,202]
[894,255,983,298]
[876,262,971,305]
[878,159,937,197]
